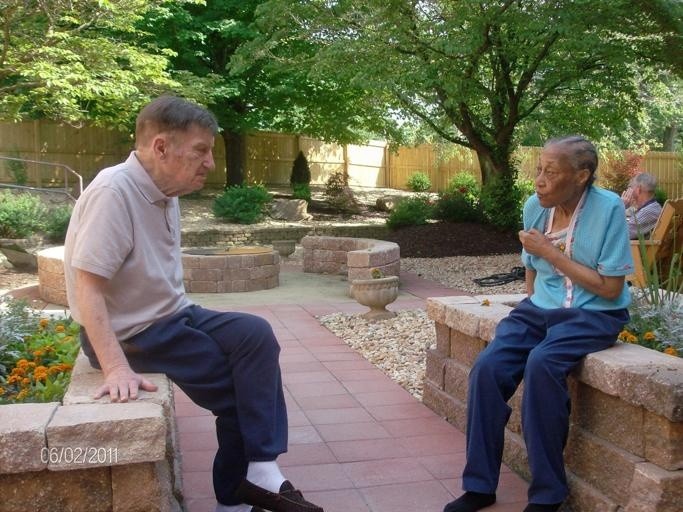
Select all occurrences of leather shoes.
[234,477,324,512]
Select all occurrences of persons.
[64,96,323,512]
[444,135,636,512]
[621,173,662,241]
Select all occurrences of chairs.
[625,198,683,289]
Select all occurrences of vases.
[350,276,399,322]
[270,240,296,262]
